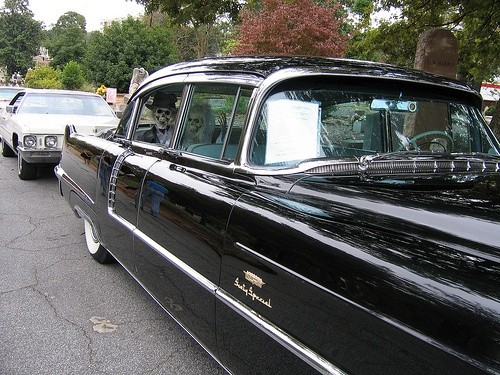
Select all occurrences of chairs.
[187,142,266,165]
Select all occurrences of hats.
[144,92,178,112]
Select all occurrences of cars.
[54,55,500,375]
[0,86,121,181]
